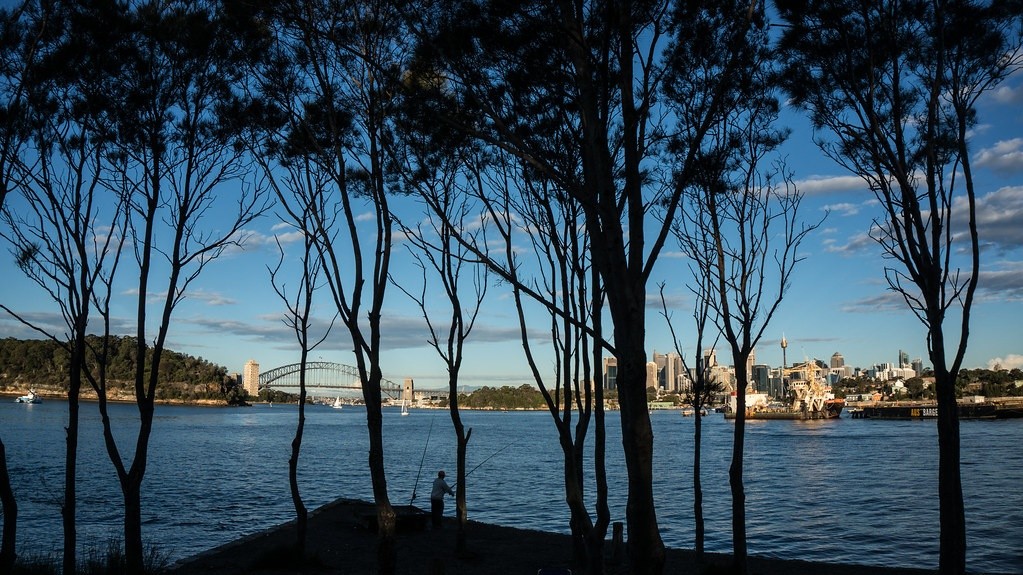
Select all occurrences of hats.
[438,471,446,476]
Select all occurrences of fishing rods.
[410,414,435,505]
[445,436,525,495]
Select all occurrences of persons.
[431,471,454,528]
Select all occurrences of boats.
[723,345,845,420]
[847,395,997,420]
[15,384,42,404]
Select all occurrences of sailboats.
[333,396,343,409]
[401,398,410,416]
